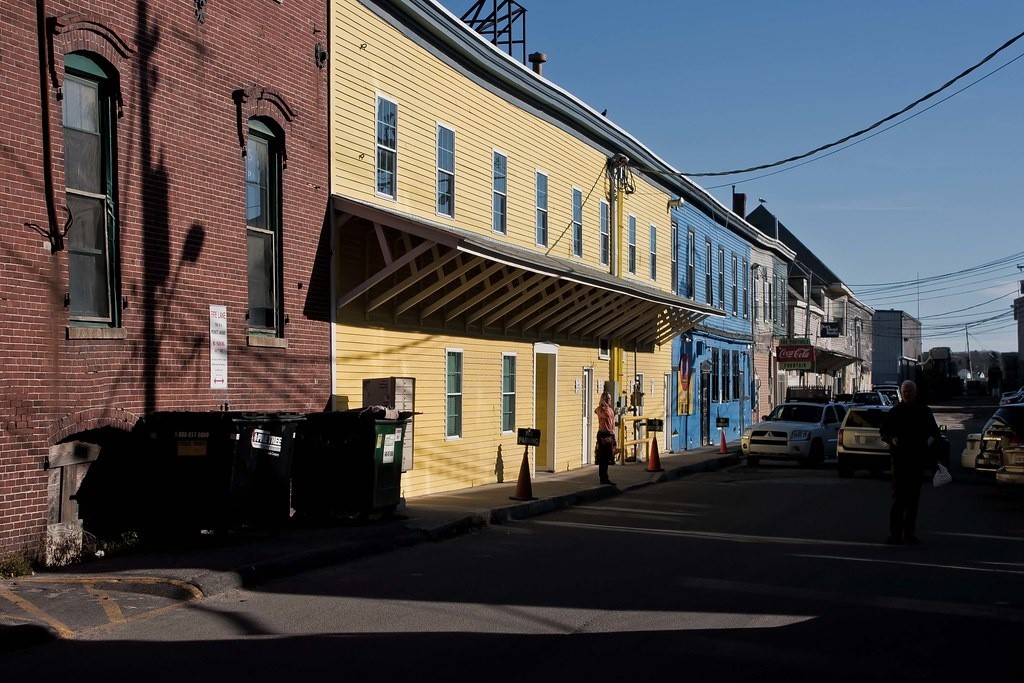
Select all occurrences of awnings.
[814,345,861,377]
[326,194,727,348]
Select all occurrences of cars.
[961,433,981,469]
[1000,386,1024,405]
[996,444,1024,489]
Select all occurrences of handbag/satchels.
[602,437,612,446]
[932,464,952,489]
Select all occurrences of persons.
[987,361,1003,399]
[594,391,619,485]
[878,379,940,543]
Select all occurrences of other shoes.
[600,480,617,486]
[906,535,922,546]
[886,533,904,545]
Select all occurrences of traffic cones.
[645,437,664,472]
[717,431,733,454]
[508,448,538,501]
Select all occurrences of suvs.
[836,405,897,476]
[974,403,1024,472]
[740,402,847,469]
[851,385,902,407]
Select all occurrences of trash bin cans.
[145,410,307,534]
[305,404,412,521]
